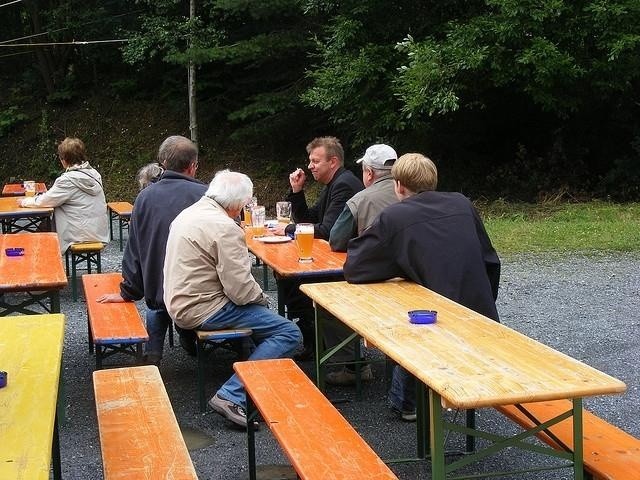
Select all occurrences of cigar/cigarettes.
[296,168,298,170]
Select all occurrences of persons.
[136,163,164,190]
[273,136,365,361]
[344,153,500,422]
[17,137,110,256]
[96,136,244,366]
[323,144,398,386]
[163,169,303,431]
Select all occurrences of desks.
[239,215,366,402]
[0,196,55,234]
[2,182,48,233]
[0,313,65,480]
[298,277,627,480]
[0,231,69,313]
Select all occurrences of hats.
[354,142,398,169]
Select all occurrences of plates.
[259,236,292,243]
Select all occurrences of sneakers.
[325,364,376,386]
[390,390,418,426]
[295,342,315,362]
[205,392,260,432]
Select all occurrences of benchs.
[65,240,104,302]
[93,365,200,480]
[107,201,134,252]
[82,272,150,370]
[168,301,254,413]
[234,357,400,480]
[464,375,640,480]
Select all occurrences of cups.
[294,223,315,263]
[24,181,35,197]
[276,201,292,224]
[244,198,266,239]
